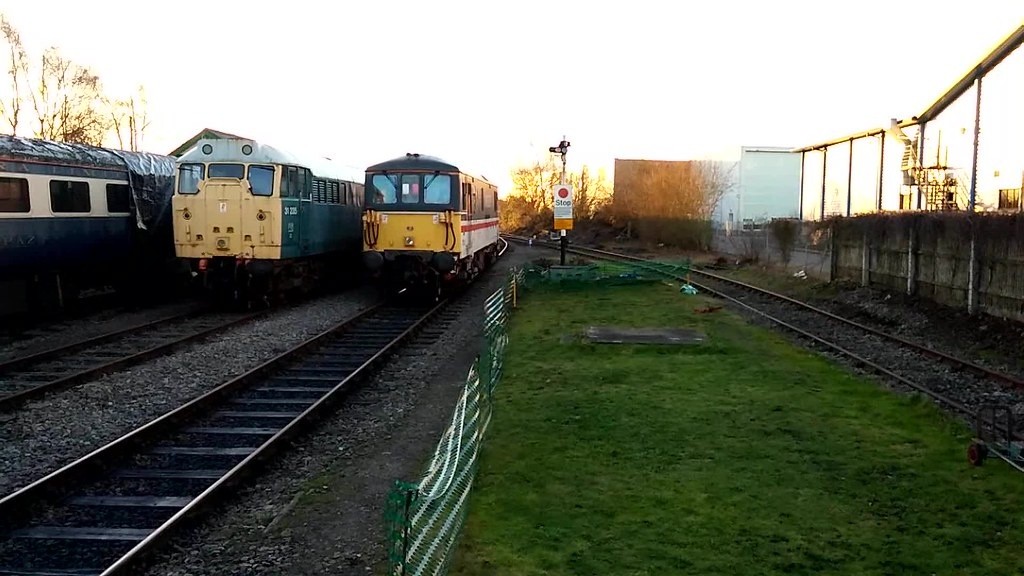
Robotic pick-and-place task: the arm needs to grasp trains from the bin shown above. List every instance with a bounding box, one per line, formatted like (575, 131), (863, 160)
(0, 133), (201, 319)
(363, 153), (500, 294)
(169, 138), (366, 312)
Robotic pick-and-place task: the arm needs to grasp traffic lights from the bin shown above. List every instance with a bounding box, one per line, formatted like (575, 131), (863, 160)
(550, 147), (568, 154)
